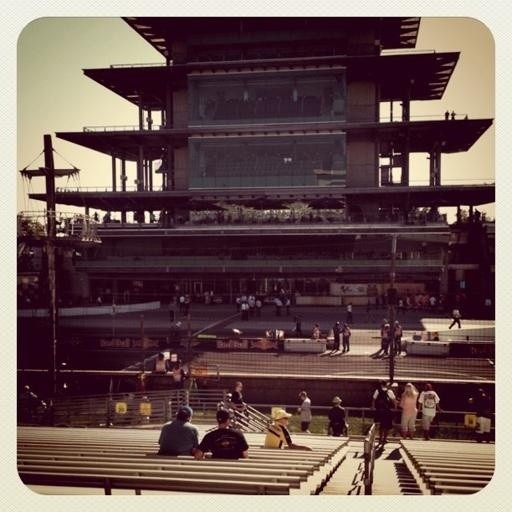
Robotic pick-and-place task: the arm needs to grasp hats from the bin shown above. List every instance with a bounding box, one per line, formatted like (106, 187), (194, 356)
(216, 410), (234, 421)
(178, 406), (193, 419)
(332, 396), (343, 403)
(274, 410), (292, 420)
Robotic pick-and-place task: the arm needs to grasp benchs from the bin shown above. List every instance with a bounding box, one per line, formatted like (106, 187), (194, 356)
(17, 424), (495, 496)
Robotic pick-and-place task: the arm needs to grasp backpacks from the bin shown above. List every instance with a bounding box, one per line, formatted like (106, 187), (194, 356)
(375, 386), (395, 412)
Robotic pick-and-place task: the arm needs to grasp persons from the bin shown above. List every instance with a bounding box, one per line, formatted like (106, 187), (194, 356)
(169, 294), (192, 321)
(328, 395), (349, 436)
(449, 305), (461, 329)
(372, 379), (443, 444)
(236, 294), (291, 321)
(346, 302), (352, 324)
(156, 353), (188, 389)
(312, 324), (320, 339)
(265, 409), (293, 450)
(223, 382), (247, 430)
(376, 294), (443, 314)
(377, 318), (402, 354)
(265, 329), (286, 339)
(156, 405), (199, 456)
(463, 388), (491, 443)
(333, 321), (350, 352)
(194, 410), (249, 460)
(297, 391), (313, 433)
